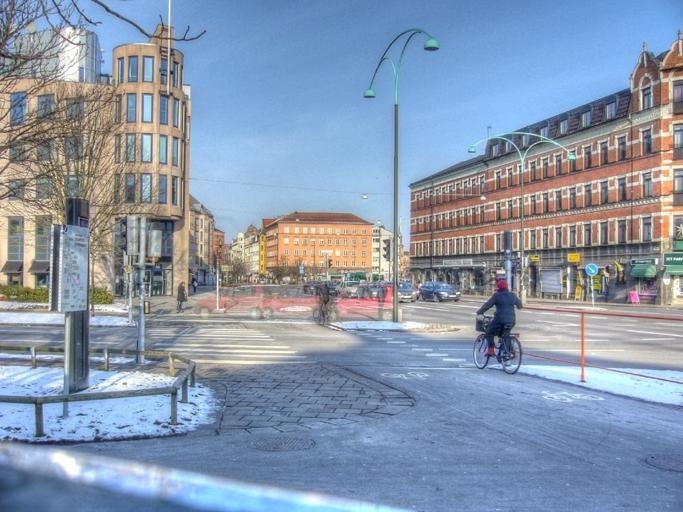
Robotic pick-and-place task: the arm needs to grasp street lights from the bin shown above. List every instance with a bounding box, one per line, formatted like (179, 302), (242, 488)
(467, 132), (576, 304)
(362, 27), (440, 322)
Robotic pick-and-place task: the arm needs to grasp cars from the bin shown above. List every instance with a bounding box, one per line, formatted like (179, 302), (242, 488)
(283, 276), (296, 284)
(304, 281), (461, 303)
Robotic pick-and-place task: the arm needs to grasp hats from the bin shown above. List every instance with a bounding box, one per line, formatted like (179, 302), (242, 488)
(497, 281), (509, 288)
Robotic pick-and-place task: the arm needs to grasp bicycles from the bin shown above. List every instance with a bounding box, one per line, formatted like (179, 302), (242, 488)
(473, 314), (522, 374)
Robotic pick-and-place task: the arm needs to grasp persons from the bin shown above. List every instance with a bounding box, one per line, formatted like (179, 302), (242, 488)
(477, 280), (522, 367)
(191, 281), (197, 292)
(175, 281), (186, 313)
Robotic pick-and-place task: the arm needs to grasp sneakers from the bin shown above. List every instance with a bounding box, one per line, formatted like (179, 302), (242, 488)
(484, 346), (497, 356)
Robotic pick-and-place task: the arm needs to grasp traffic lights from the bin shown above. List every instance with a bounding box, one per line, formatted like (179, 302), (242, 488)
(382, 239), (390, 261)
(329, 259), (332, 268)
